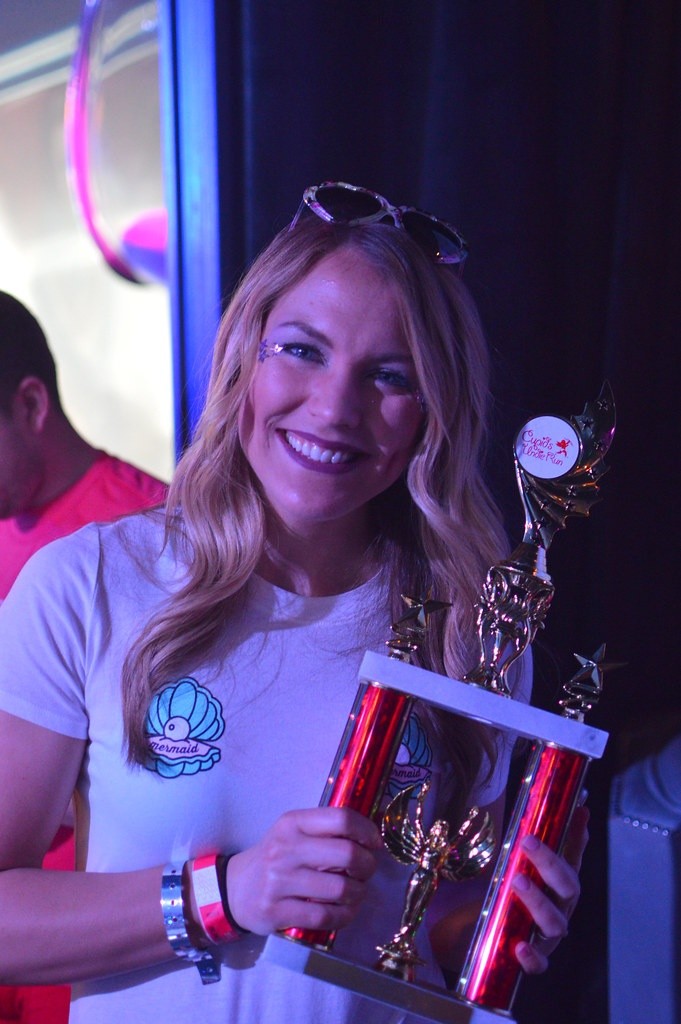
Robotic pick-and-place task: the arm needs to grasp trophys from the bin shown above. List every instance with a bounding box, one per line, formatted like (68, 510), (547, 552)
(256, 386), (619, 1023)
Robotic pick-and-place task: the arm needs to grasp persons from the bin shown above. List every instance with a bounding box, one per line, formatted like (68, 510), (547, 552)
(0, 292), (174, 1023)
(0, 178), (583, 1023)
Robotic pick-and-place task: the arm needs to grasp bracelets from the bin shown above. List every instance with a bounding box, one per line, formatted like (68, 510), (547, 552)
(160, 858), (225, 985)
(191, 839), (250, 948)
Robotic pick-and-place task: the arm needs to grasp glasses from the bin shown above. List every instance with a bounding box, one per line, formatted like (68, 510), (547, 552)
(290, 181), (467, 274)
